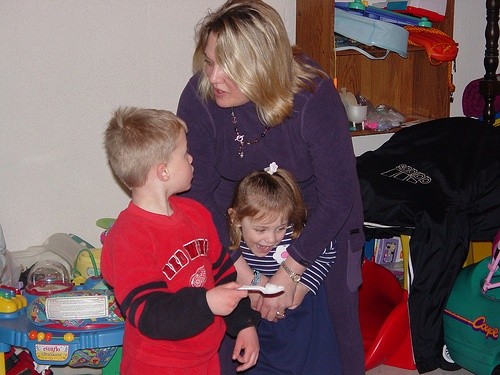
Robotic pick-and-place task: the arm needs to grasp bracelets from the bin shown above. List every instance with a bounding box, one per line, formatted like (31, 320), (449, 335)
(251, 269), (260, 286)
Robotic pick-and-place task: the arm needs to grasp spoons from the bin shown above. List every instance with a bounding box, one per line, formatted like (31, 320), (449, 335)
(237, 284), (284, 294)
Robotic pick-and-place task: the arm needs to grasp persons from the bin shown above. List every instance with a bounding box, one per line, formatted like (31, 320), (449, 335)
(99, 105), (261, 375)
(228, 162), (344, 375)
(177, 0), (365, 375)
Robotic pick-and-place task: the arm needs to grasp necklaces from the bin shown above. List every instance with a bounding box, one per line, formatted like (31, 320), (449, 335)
(229, 105), (269, 159)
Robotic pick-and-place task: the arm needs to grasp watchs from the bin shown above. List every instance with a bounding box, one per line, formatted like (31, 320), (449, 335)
(281, 261), (301, 283)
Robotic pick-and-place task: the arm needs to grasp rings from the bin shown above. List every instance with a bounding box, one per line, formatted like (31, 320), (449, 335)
(282, 314), (286, 318)
(276, 311), (281, 317)
